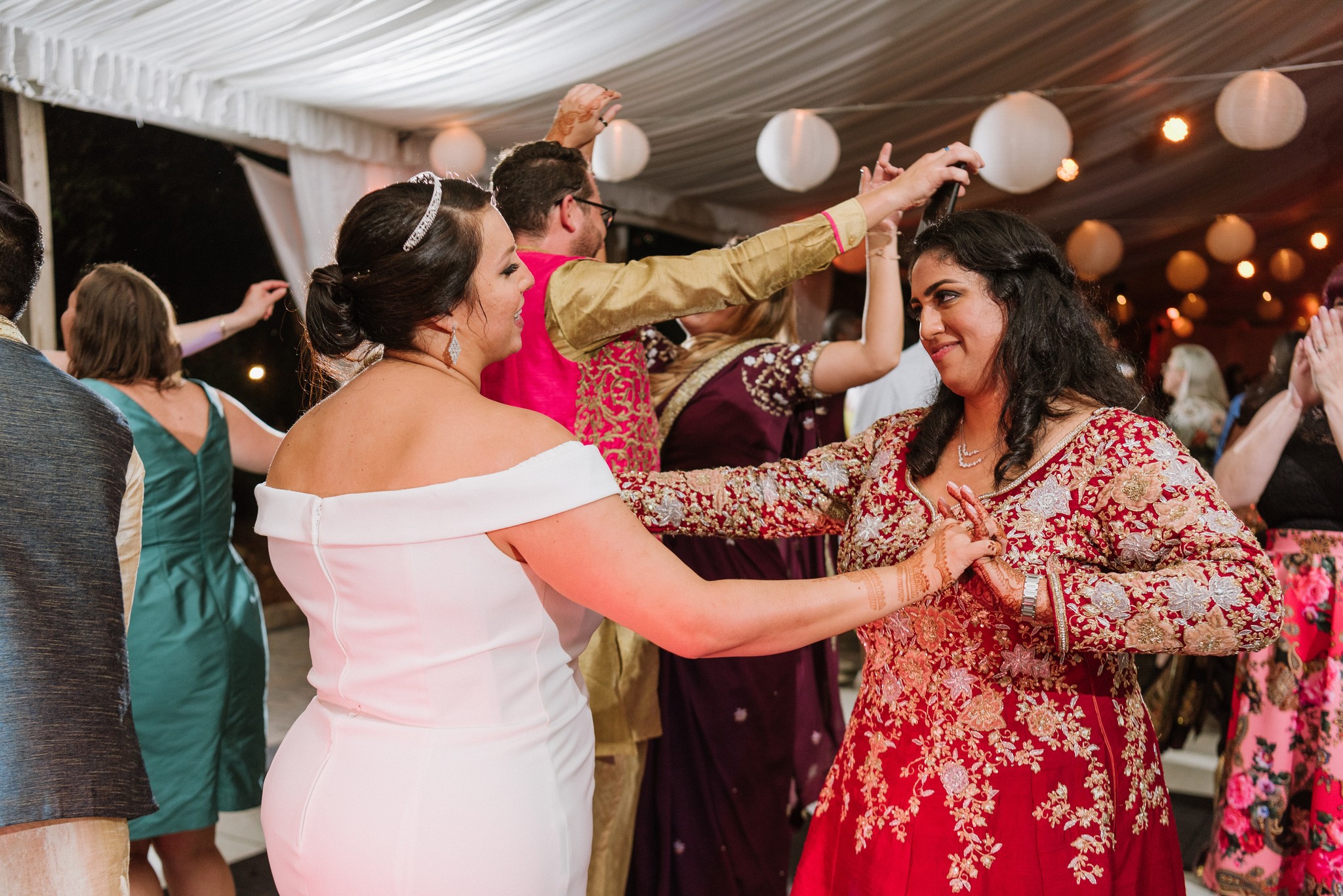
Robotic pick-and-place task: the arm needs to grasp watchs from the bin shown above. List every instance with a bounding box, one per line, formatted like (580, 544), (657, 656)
(1020, 573), (1044, 622)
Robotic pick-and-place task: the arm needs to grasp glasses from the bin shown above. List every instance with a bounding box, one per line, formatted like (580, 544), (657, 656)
(555, 194), (617, 230)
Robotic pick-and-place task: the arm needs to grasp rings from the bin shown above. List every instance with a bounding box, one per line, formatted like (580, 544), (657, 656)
(599, 117), (608, 127)
(602, 86), (608, 92)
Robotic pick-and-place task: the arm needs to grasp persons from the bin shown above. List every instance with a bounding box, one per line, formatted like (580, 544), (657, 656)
(577, 143), (904, 896)
(1205, 264), (1343, 896)
(253, 172), (1003, 896)
(477, 83), (983, 896)
(59, 261), (289, 896)
(616, 213), (1284, 895)
(822, 309), (1305, 448)
(1, 182), (159, 896)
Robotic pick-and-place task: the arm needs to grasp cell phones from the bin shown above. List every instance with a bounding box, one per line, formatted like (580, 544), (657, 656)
(916, 163), (967, 243)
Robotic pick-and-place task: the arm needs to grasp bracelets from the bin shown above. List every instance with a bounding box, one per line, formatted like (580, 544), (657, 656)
(219, 314), (227, 340)
(867, 231), (902, 237)
(865, 247), (902, 260)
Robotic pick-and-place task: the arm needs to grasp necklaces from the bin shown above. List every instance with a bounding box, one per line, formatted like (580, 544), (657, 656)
(959, 411), (1006, 468)
